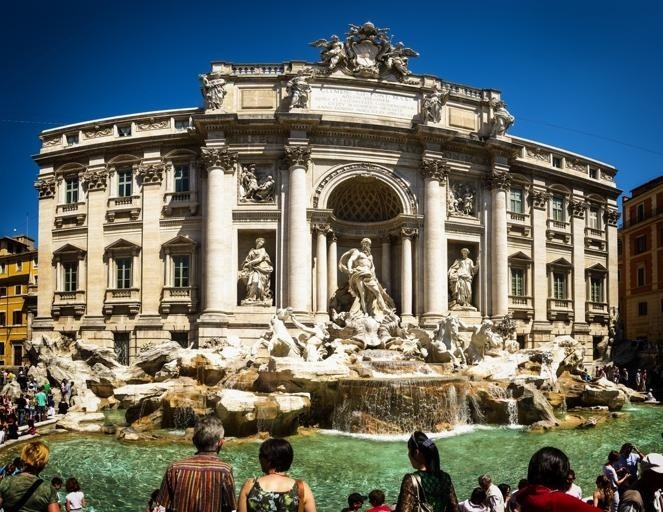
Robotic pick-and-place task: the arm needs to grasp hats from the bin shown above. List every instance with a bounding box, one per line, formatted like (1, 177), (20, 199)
(348, 493), (369, 502)
(641, 452), (663, 473)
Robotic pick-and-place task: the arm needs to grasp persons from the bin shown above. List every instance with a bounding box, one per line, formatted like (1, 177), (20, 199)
(238, 236), (274, 301)
(238, 437), (317, 512)
(146, 490), (161, 511)
(447, 247), (478, 306)
(1, 365), (87, 512)
(238, 164), (275, 200)
(153, 415), (237, 512)
(448, 182), (476, 216)
(338, 237), (391, 316)
(595, 363), (647, 393)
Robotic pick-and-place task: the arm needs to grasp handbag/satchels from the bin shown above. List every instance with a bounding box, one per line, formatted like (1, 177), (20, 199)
(409, 472), (433, 511)
